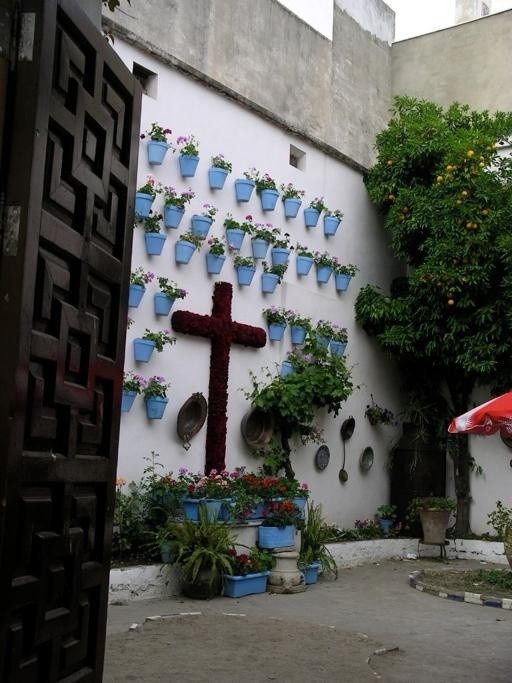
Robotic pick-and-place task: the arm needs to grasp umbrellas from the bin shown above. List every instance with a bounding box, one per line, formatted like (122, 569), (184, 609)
(448, 390), (512, 433)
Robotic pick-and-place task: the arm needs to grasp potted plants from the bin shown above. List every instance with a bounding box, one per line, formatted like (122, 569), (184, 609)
(142, 501), (251, 601)
(409, 496), (454, 543)
(375, 504), (397, 535)
